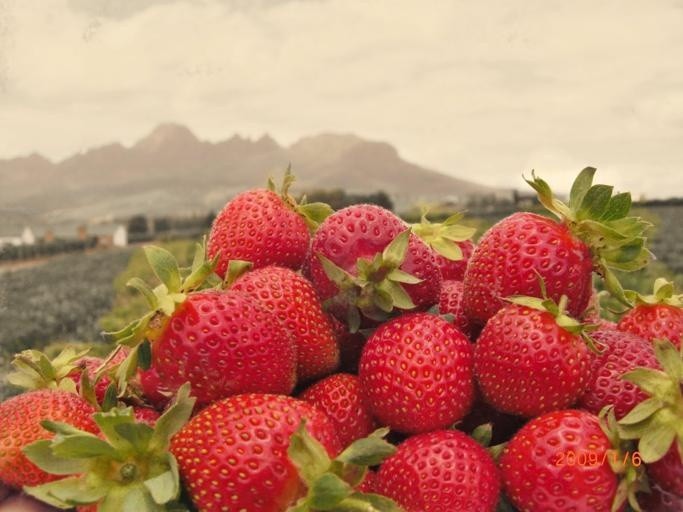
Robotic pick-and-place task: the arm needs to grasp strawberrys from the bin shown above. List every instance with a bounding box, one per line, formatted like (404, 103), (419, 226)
(0, 166), (683, 512)
